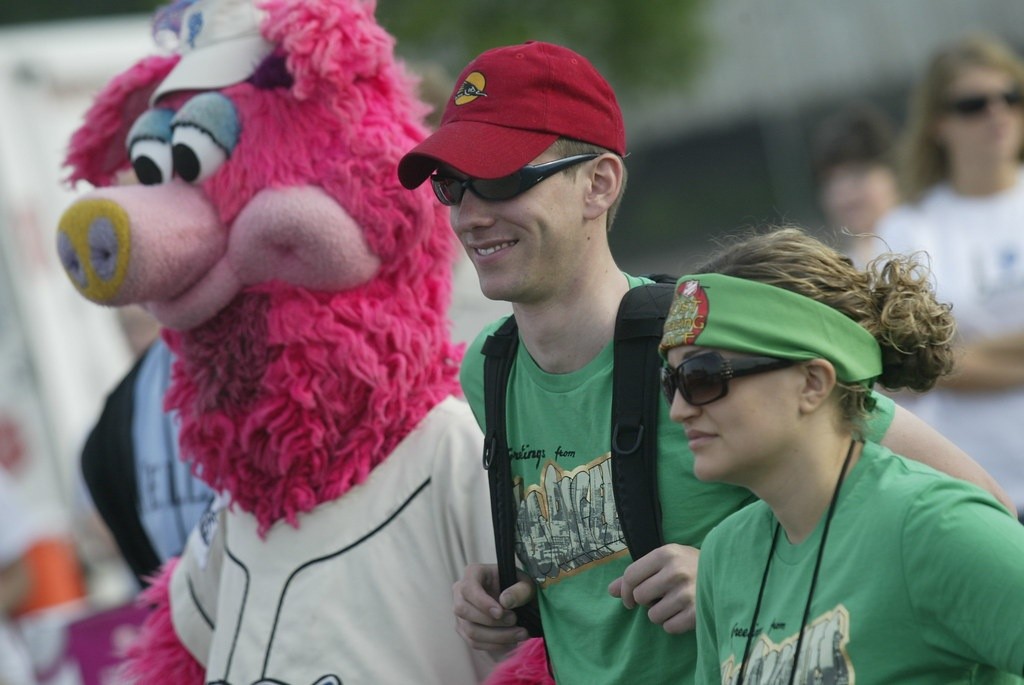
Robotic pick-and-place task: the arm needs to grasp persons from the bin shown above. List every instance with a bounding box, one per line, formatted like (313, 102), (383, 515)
(658, 227), (1024, 685)
(859, 30), (1024, 519)
(808, 118), (898, 240)
(399, 41), (1019, 685)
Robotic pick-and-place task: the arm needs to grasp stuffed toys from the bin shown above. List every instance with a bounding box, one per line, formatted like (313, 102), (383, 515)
(57, 0), (498, 685)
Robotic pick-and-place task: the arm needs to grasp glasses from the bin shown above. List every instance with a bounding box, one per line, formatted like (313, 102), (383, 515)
(664, 350), (795, 406)
(429, 152), (600, 207)
(933, 93), (1024, 118)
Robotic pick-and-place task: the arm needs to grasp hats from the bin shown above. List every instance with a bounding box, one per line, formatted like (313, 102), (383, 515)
(397, 40), (626, 190)
